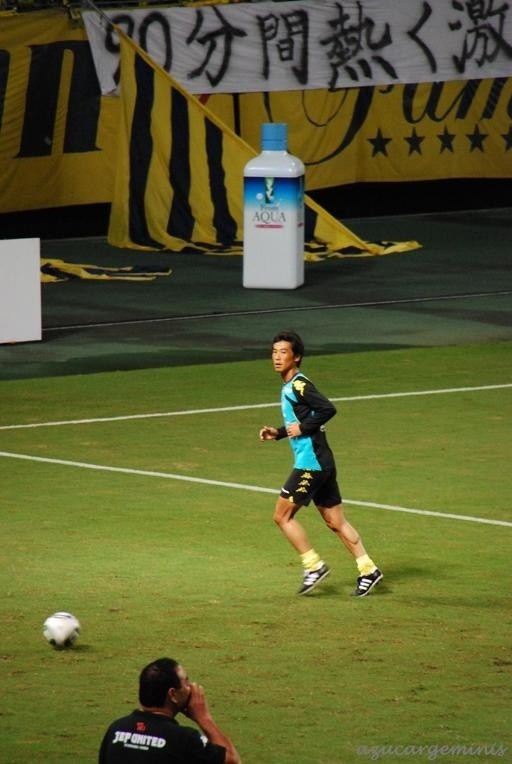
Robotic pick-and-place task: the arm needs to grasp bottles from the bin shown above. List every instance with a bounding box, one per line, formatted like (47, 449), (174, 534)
(241, 121), (306, 293)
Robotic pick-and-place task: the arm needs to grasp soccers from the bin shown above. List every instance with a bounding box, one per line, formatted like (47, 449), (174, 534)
(42, 612), (81, 649)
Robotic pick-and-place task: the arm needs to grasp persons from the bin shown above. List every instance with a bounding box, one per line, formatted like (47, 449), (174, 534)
(97, 655), (246, 764)
(257, 327), (385, 597)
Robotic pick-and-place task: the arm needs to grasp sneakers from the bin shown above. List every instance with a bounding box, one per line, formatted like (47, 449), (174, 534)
(296, 563), (331, 595)
(349, 568), (385, 597)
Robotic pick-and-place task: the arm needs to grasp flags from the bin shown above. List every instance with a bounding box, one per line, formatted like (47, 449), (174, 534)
(104, 24), (423, 267)
